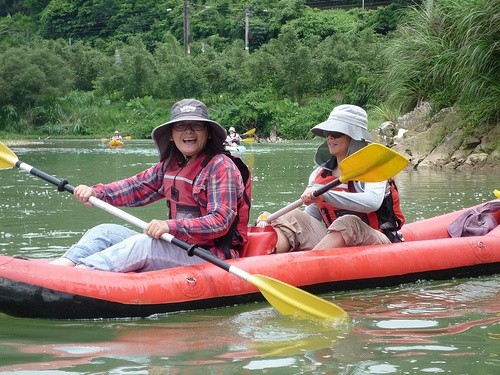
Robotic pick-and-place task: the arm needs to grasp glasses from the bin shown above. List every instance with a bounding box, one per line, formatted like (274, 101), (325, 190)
(322, 130), (345, 138)
(174, 121), (204, 131)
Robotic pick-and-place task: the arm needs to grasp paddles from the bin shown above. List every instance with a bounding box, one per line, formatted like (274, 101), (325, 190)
(101, 135), (132, 143)
(266, 143), (409, 223)
(0, 143), (351, 325)
(223, 128), (256, 145)
(225, 138), (254, 143)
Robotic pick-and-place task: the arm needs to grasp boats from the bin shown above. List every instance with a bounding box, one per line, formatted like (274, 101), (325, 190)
(224, 145), (247, 154)
(108, 139), (124, 148)
(0, 189), (500, 319)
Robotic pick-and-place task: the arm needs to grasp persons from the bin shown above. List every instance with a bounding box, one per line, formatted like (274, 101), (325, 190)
(47, 98), (253, 272)
(223, 127), (242, 145)
(111, 130), (124, 143)
(265, 104), (405, 253)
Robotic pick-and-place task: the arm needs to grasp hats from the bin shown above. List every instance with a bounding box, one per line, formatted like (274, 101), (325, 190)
(228, 127), (236, 132)
(115, 131), (119, 133)
(310, 105), (373, 144)
(151, 99), (227, 152)
